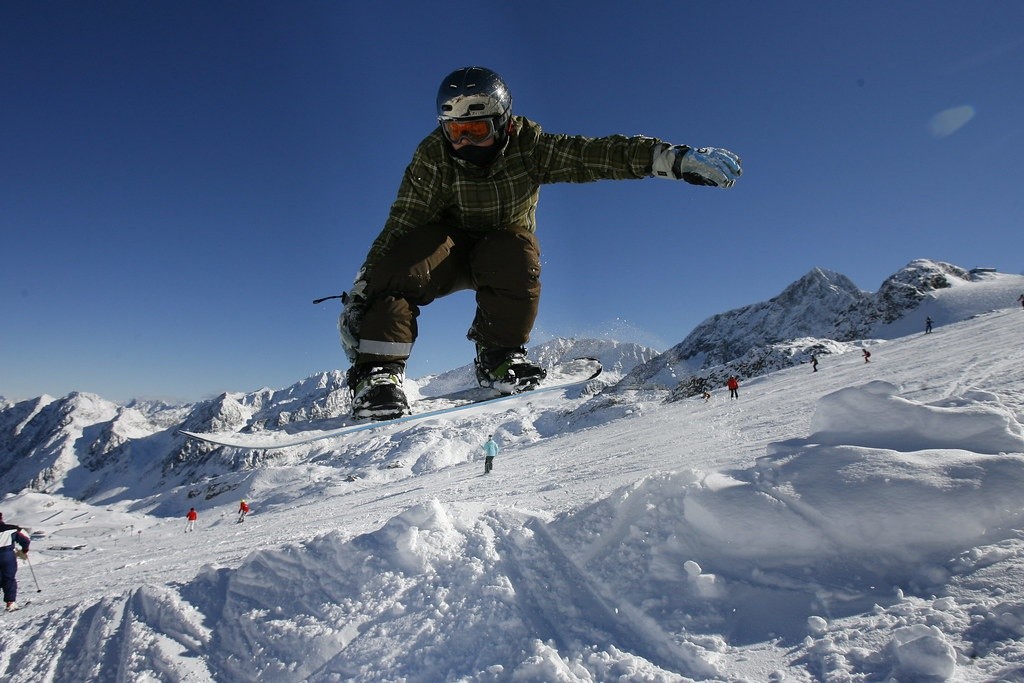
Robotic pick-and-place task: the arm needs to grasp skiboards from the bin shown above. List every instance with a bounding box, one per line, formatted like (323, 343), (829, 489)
(0, 601), (31, 616)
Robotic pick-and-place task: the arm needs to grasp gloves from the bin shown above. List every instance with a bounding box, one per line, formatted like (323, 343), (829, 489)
(651, 142), (743, 188)
(338, 283), (365, 364)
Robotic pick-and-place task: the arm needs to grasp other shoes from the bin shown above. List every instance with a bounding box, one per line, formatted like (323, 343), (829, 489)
(7, 602), (18, 609)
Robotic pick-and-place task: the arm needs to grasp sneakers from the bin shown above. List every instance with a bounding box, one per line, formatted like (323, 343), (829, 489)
(474, 345), (546, 385)
(347, 366), (406, 419)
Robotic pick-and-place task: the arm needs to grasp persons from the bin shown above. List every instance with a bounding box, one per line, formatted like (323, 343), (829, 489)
(237, 499), (248, 523)
(480, 434), (498, 475)
(184, 507), (197, 533)
(701, 316), (934, 402)
(1017, 294), (1024, 310)
(339, 68), (743, 421)
(0, 512), (31, 611)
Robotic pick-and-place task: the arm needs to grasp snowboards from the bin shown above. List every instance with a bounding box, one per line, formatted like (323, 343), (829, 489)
(176, 356), (604, 448)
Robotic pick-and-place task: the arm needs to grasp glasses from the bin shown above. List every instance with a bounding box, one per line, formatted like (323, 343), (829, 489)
(438, 97), (511, 146)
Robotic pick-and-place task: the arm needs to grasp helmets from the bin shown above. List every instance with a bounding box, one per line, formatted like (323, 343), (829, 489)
(241, 499), (246, 502)
(436, 67), (513, 182)
